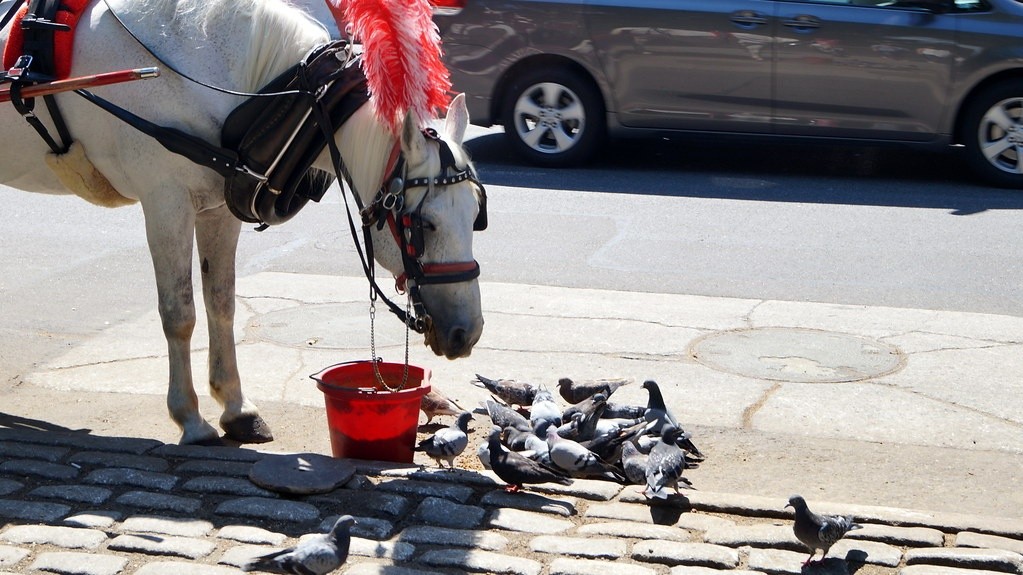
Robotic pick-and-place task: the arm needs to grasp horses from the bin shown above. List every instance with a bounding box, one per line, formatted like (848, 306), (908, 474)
(0, 0), (485, 448)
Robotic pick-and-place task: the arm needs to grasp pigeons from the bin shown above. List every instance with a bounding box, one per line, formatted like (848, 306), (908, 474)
(411, 373), (707, 498)
(217, 515), (359, 575)
(784, 495), (863, 570)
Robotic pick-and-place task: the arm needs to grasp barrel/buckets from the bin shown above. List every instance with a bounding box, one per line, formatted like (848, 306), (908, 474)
(309, 356), (432, 463)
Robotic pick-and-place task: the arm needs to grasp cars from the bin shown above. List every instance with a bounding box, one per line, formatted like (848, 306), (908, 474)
(423, 0), (1022, 191)
